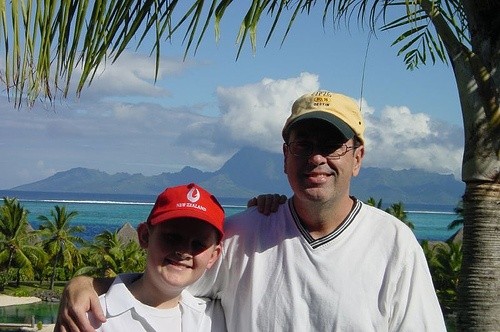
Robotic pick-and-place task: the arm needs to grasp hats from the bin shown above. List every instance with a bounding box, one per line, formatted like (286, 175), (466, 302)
(282, 90), (365, 145)
(149, 183), (225, 245)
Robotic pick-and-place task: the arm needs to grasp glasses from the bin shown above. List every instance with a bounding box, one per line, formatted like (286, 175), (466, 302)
(285, 142), (357, 156)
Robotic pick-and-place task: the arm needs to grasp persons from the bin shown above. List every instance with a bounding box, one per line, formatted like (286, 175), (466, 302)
(53, 88), (448, 332)
(78, 180), (288, 332)
